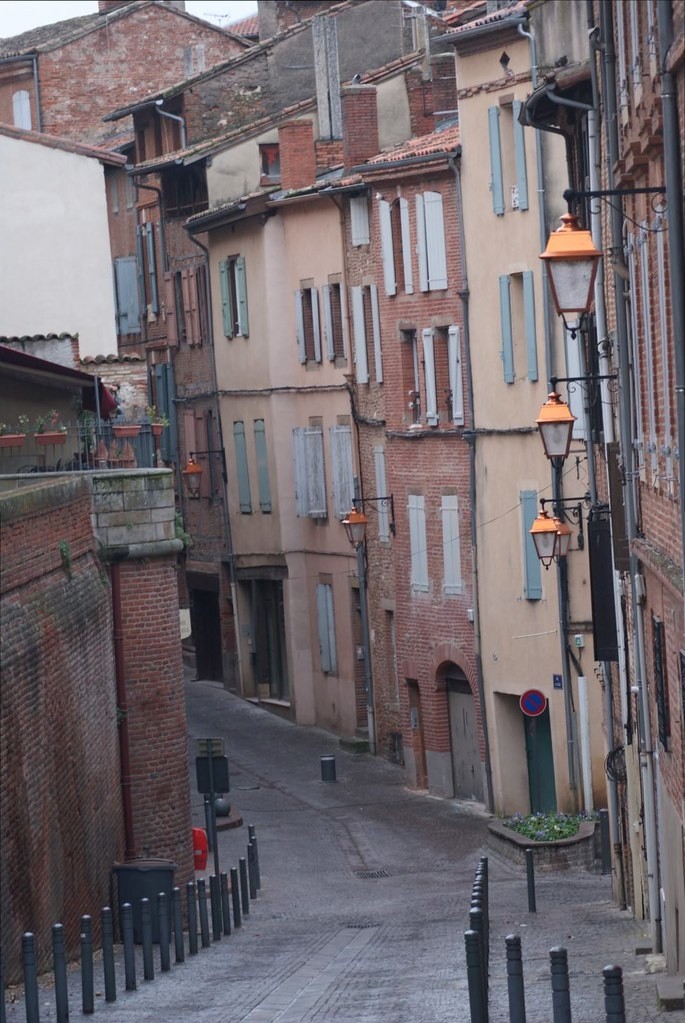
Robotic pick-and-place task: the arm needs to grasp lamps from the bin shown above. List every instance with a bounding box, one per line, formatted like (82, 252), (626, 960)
(535, 369), (615, 468)
(182, 449), (225, 498)
(532, 495), (591, 568)
(541, 187), (665, 339)
(340, 494), (393, 551)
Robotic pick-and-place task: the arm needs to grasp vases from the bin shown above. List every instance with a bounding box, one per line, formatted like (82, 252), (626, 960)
(33, 432), (67, 445)
(0, 434), (26, 447)
(111, 425), (140, 437)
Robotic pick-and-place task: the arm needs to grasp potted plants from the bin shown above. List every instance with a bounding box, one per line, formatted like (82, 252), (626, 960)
(148, 407), (170, 434)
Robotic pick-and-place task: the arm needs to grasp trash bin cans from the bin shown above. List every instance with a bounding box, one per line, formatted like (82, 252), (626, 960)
(113, 860), (179, 944)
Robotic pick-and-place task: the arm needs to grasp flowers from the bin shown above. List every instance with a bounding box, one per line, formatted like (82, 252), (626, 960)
(0, 414), (30, 436)
(35, 410), (66, 434)
